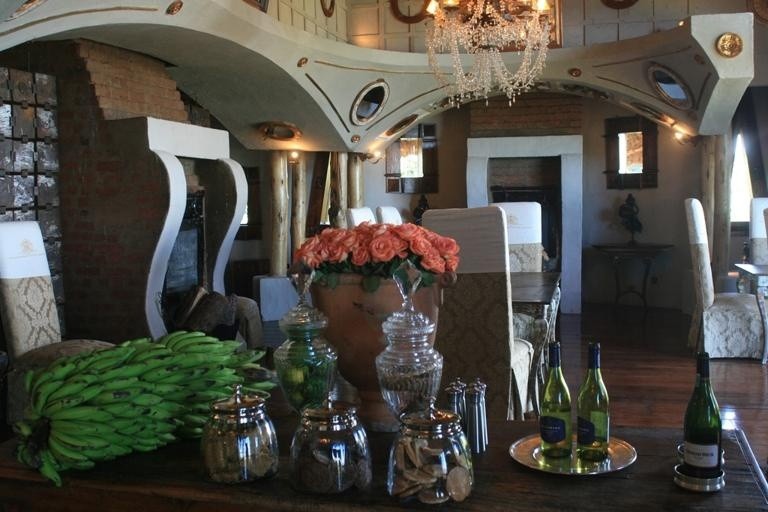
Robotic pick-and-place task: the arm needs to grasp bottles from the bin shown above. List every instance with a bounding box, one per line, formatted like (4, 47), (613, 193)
(449, 376), (467, 418)
(683, 352), (722, 478)
(465, 383), (487, 457)
(468, 376), (489, 448)
(576, 341), (611, 462)
(539, 341), (573, 459)
(445, 381), (468, 440)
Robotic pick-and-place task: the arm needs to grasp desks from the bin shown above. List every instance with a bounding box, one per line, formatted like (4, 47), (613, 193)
(0, 425), (768, 512)
(592, 241), (676, 315)
(732, 261), (768, 275)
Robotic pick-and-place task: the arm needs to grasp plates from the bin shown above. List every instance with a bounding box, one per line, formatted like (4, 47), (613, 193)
(508, 428), (638, 477)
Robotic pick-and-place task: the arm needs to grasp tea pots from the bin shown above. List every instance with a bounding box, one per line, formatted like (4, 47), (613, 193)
(292, 398), (371, 495)
(204, 384), (277, 483)
(389, 395), (471, 506)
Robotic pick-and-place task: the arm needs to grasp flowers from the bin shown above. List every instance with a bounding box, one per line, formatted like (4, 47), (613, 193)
(292, 222), (460, 275)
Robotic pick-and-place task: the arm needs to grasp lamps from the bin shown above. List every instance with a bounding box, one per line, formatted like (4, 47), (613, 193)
(424, 1), (551, 106)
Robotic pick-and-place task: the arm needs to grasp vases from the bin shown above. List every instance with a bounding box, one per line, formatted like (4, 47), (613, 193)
(310, 275), (458, 422)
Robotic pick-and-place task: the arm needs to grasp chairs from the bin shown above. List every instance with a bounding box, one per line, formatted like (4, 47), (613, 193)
(378, 205), (404, 225)
(684, 197), (763, 361)
(490, 199), (559, 313)
(749, 197), (768, 365)
(345, 205), (377, 227)
(422, 206), (539, 422)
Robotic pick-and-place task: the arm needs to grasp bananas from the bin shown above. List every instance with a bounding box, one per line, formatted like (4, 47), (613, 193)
(11, 328), (281, 488)
